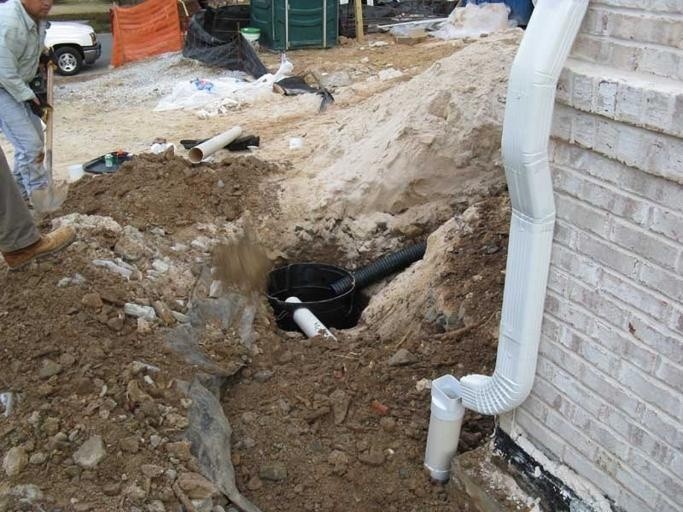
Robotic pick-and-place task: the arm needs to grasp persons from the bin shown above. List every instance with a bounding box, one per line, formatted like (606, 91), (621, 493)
(0, 143), (76, 270)
(0, 0), (54, 208)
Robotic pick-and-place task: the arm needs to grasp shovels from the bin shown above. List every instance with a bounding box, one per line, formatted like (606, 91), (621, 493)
(31, 66), (69, 215)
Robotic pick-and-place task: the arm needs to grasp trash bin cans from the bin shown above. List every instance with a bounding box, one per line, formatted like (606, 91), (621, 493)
(240, 27), (261, 54)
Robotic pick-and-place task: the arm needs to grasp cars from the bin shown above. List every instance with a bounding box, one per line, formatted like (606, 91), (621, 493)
(39, 19), (102, 76)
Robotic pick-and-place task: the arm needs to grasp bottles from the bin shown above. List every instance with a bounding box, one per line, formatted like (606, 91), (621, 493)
(105, 153), (114, 168)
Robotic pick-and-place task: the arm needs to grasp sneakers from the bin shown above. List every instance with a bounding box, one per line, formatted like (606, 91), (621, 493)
(3, 226), (76, 267)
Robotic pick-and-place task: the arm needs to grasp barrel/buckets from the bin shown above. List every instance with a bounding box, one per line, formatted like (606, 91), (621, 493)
(241, 28), (261, 43)
